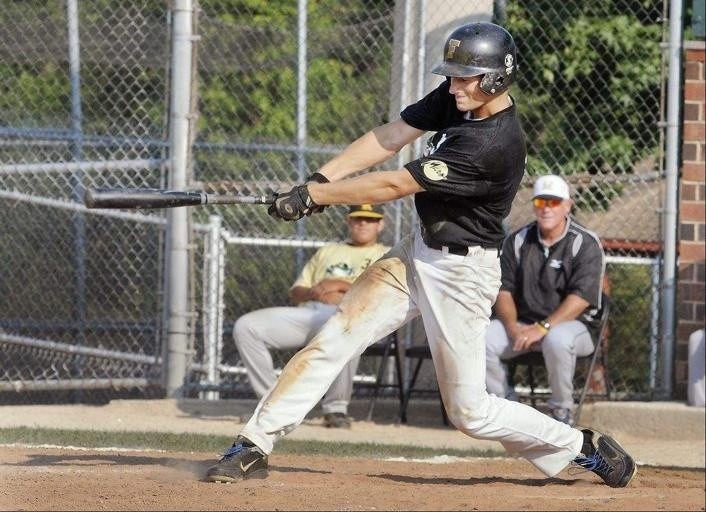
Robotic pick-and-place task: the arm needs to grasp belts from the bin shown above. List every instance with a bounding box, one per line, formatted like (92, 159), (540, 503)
(420, 223), (468, 256)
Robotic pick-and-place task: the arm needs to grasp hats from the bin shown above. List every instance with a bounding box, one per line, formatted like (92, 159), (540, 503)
(531, 174), (570, 201)
(347, 203), (384, 219)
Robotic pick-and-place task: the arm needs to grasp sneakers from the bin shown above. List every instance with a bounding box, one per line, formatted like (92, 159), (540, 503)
(322, 413), (350, 428)
(573, 427), (637, 487)
(206, 438), (269, 484)
(547, 408), (576, 427)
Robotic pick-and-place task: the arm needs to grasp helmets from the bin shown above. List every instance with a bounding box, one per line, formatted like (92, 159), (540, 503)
(431, 21), (516, 96)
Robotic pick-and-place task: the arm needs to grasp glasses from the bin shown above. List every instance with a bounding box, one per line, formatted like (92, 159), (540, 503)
(532, 197), (562, 207)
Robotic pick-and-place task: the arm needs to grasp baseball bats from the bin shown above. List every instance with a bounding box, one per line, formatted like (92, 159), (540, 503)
(84, 188), (280, 209)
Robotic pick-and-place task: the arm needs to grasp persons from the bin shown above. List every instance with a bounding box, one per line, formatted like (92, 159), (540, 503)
(233, 200), (397, 429)
(481, 175), (609, 427)
(205, 22), (638, 487)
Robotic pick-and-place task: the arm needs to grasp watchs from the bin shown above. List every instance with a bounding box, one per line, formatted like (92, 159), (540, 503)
(534, 317), (550, 334)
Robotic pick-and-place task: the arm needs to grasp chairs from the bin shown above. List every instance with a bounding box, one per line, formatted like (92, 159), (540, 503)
(502, 292), (612, 427)
(350, 329), (407, 425)
(396, 337), (450, 427)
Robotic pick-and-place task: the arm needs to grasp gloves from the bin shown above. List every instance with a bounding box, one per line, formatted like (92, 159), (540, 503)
(268, 173), (331, 221)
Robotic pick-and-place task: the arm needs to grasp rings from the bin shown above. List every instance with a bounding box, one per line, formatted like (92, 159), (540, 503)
(523, 335), (529, 341)
